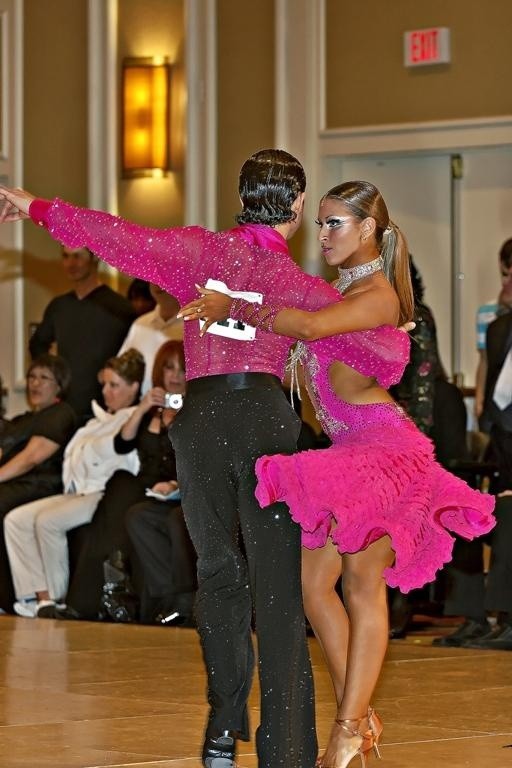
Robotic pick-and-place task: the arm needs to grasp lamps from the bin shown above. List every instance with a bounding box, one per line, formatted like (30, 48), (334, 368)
(119, 55), (172, 177)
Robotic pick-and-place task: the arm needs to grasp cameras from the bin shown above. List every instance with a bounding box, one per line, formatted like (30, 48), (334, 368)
(162, 392), (184, 411)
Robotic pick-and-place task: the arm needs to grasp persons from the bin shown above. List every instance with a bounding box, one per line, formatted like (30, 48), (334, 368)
(174, 179), (499, 767)
(0, 233), (512, 650)
(0, 146), (415, 768)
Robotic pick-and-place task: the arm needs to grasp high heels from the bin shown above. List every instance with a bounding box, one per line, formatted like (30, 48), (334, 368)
(366, 707), (384, 758)
(317, 717), (375, 767)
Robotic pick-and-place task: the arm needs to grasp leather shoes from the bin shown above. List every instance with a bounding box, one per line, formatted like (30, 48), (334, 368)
(464, 623), (512, 649)
(432, 619), (491, 646)
(203, 725), (237, 768)
(13, 600), (66, 617)
